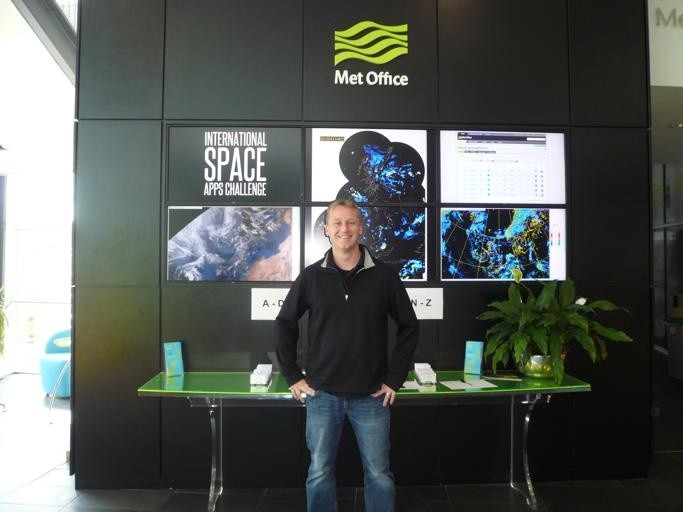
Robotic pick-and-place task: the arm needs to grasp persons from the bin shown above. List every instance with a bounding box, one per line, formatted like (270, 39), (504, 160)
(275, 199), (418, 508)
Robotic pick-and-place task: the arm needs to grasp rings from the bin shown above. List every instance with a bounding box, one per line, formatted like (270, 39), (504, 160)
(386, 393), (390, 395)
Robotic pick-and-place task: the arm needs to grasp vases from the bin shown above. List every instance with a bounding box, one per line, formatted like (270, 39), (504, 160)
(512, 350), (566, 379)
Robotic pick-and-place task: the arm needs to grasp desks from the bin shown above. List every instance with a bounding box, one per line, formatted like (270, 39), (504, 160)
(136, 369), (592, 512)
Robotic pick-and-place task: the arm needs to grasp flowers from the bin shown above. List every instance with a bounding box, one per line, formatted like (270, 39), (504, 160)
(475, 268), (634, 386)
